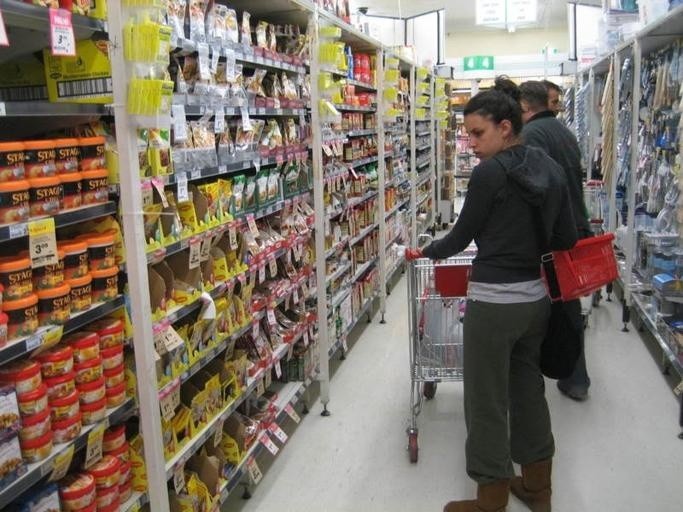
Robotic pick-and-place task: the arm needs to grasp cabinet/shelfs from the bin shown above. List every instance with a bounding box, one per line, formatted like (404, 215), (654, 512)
(0, 0), (457, 512)
(558, 5), (683, 426)
(449, 87), (480, 197)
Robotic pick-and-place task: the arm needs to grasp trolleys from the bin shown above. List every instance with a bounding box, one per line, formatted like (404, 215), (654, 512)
(407, 234), (479, 463)
(580, 181), (605, 329)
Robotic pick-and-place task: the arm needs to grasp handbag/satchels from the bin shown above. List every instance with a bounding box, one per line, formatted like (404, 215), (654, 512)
(539, 301), (585, 380)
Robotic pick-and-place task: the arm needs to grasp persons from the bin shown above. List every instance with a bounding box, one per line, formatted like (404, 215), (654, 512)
(414, 74), (580, 512)
(540, 78), (562, 117)
(514, 81), (593, 400)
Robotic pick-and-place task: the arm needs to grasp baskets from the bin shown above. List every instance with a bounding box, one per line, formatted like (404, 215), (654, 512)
(548, 233), (621, 301)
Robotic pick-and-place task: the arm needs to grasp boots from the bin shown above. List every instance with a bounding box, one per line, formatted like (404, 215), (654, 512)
(441, 480), (510, 512)
(510, 456), (556, 512)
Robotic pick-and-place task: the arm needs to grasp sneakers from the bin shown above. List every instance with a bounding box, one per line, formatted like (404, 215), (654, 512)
(556, 377), (592, 405)
